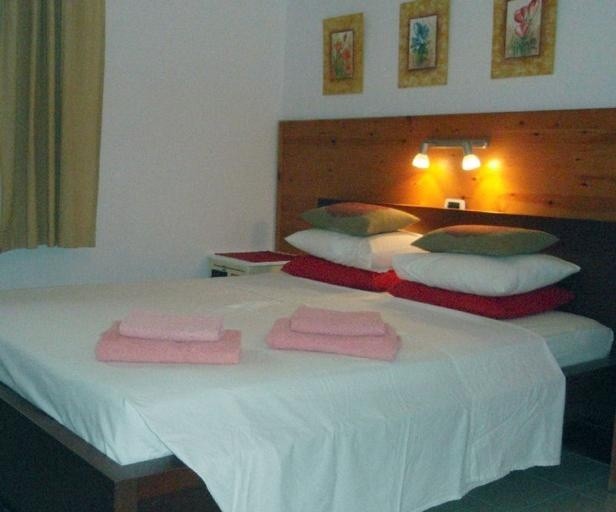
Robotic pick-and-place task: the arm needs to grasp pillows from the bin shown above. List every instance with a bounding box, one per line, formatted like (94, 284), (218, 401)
(300, 200), (421, 237)
(412, 224), (560, 258)
(281, 254), (395, 293)
(388, 276), (582, 319)
(390, 249), (582, 298)
(283, 228), (426, 273)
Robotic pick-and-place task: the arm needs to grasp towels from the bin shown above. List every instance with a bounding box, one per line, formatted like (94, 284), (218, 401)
(265, 304), (404, 363)
(93, 306), (244, 367)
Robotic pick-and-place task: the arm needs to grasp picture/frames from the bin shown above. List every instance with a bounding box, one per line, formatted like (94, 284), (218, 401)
(397, 1), (450, 89)
(490, 1), (557, 80)
(322, 11), (364, 95)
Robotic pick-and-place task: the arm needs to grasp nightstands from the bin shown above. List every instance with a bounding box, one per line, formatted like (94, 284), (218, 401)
(209, 249), (297, 276)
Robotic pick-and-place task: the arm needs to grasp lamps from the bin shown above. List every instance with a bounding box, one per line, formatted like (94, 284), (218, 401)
(412, 136), (488, 173)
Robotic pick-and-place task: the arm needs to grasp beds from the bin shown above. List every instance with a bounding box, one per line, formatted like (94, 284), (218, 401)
(1, 196), (616, 512)
